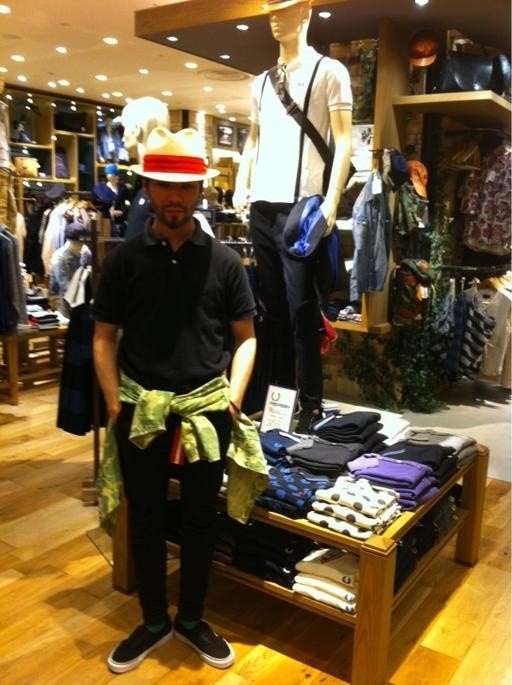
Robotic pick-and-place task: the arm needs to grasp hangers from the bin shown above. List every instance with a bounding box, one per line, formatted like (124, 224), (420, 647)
(436, 125), (512, 172)
(442, 270), (511, 308)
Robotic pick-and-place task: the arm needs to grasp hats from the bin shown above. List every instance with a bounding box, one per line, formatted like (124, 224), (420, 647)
(268, 0), (300, 12)
(104, 165), (117, 174)
(407, 160), (428, 199)
(383, 147), (410, 193)
(409, 31), (439, 67)
(45, 183), (63, 198)
(64, 222), (87, 240)
(130, 128), (221, 183)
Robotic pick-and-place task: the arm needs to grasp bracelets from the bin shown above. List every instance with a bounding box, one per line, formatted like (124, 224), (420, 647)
(228, 397), (241, 415)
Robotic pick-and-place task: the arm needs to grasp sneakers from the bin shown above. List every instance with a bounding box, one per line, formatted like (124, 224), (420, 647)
(175, 614), (235, 669)
(295, 406), (322, 434)
(108, 614), (173, 674)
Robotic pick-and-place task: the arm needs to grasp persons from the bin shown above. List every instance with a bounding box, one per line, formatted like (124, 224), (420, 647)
(93, 163), (133, 221)
(47, 223), (93, 319)
(231, 0), (353, 435)
(91, 125), (260, 676)
(121, 95), (173, 241)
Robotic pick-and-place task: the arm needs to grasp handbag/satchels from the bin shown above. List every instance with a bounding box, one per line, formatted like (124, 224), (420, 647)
(323, 154), (356, 197)
(46, 290), (60, 309)
(388, 258), (430, 327)
(393, 182), (430, 236)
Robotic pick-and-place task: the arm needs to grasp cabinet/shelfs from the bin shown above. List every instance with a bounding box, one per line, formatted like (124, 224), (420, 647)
(109, 406), (491, 685)
(202, 113), (250, 265)
(0, 84), (145, 407)
